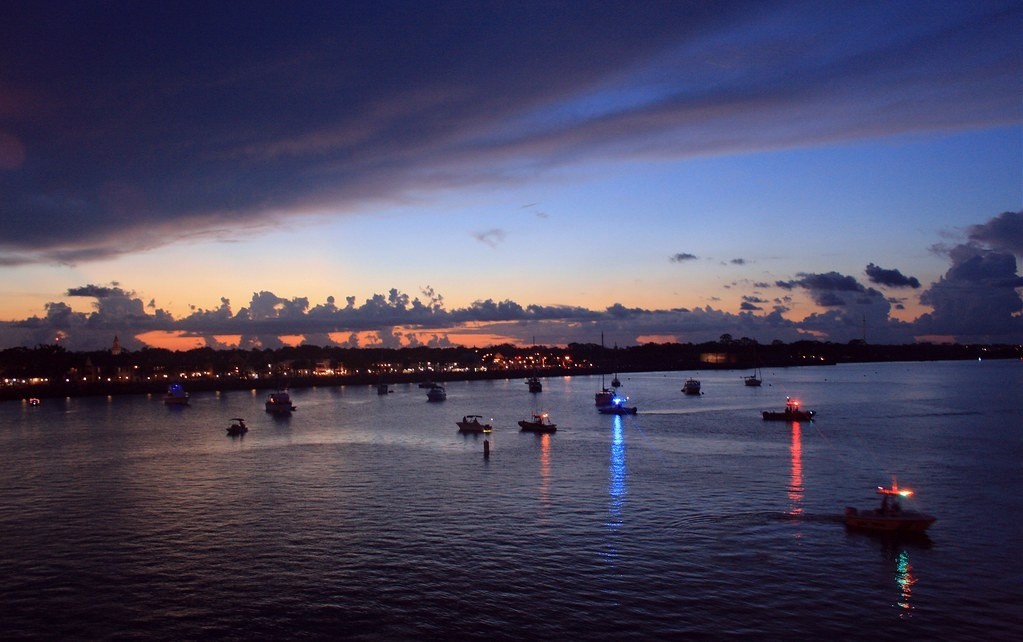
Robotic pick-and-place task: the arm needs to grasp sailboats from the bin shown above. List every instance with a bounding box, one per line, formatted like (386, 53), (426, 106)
(611, 340), (621, 387)
(522, 335), (543, 393)
(745, 336), (762, 387)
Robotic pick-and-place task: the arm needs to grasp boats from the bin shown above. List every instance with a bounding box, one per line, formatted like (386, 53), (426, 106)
(418, 382), (448, 403)
(518, 412), (557, 433)
(763, 396), (817, 423)
(378, 384), (389, 395)
(450, 412), (493, 434)
(162, 384), (190, 404)
(597, 397), (638, 415)
(226, 418), (249, 435)
(843, 493), (940, 535)
(265, 380), (293, 418)
(595, 330), (616, 406)
(681, 376), (701, 395)
